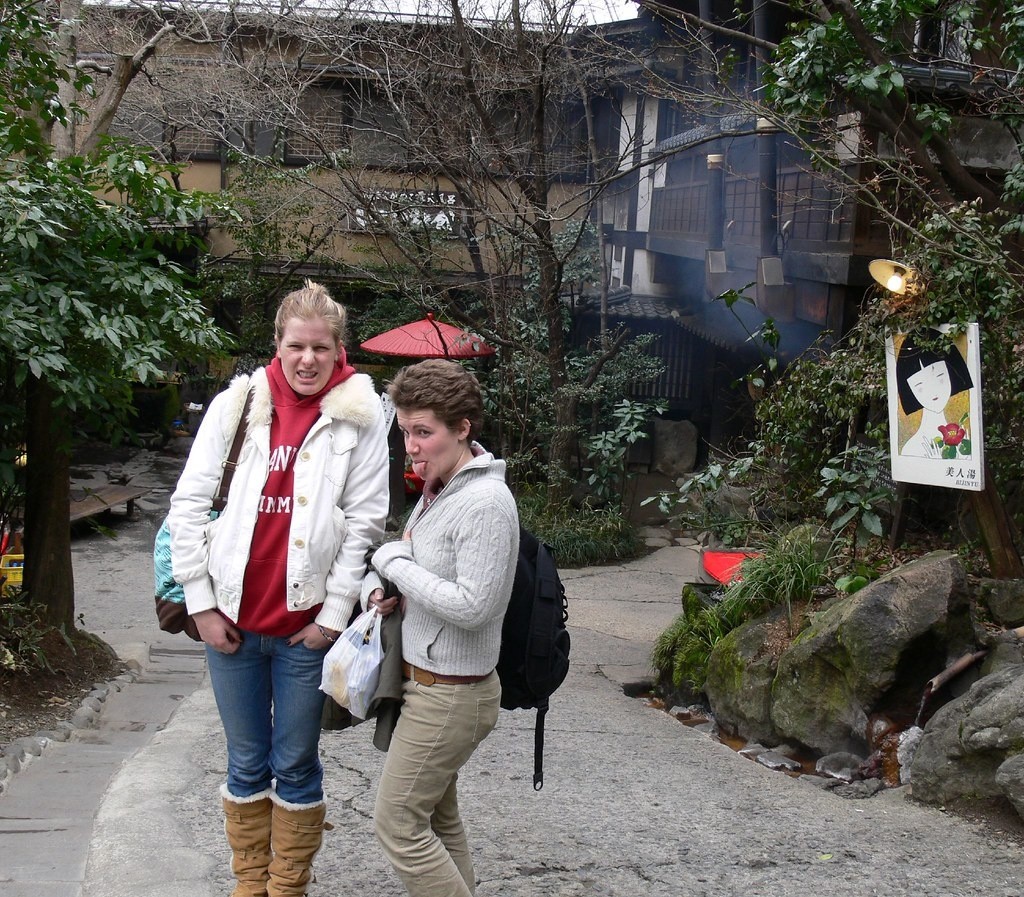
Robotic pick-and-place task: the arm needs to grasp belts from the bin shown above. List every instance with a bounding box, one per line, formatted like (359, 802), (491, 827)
(397, 660), (494, 687)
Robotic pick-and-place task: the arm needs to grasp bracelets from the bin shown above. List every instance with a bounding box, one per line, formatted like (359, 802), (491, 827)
(317, 626), (340, 643)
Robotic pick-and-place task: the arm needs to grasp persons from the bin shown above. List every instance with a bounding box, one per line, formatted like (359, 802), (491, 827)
(364, 356), (519, 897)
(167, 279), (390, 897)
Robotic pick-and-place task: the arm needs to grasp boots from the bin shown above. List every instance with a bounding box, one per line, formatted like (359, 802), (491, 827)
(220, 785), (332, 896)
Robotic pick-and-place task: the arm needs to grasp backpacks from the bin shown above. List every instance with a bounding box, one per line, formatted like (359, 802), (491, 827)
(497, 521), (571, 713)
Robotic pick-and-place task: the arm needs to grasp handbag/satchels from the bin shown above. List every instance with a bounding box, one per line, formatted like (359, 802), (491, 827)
(317, 604), (384, 722)
(155, 512), (218, 643)
